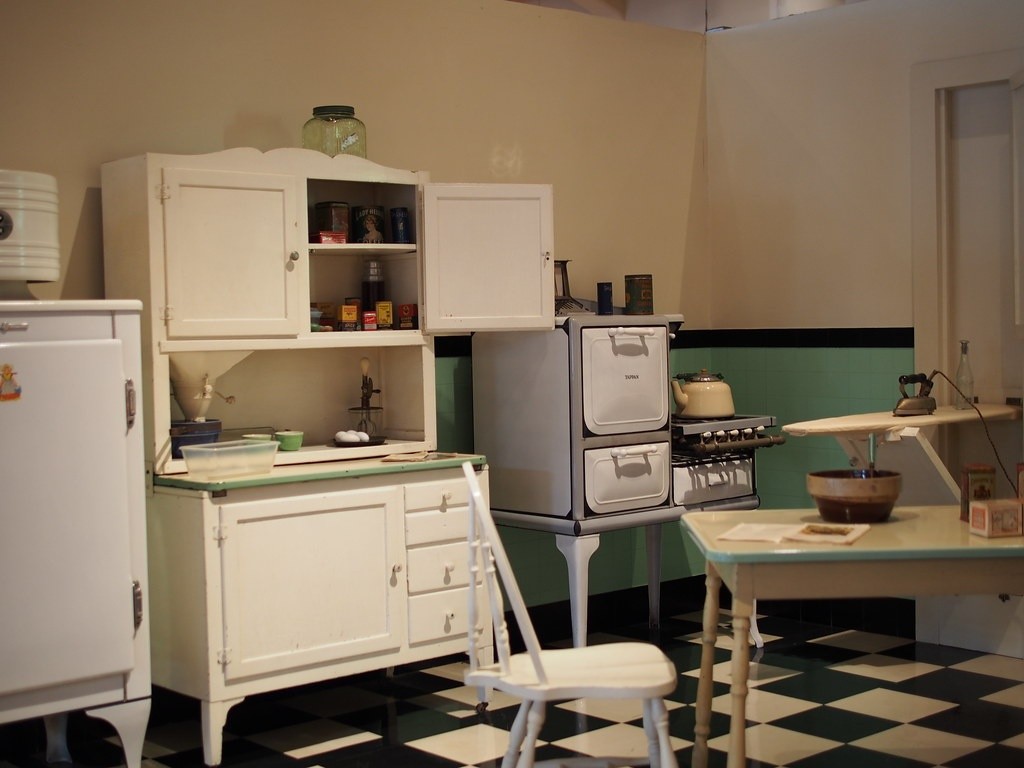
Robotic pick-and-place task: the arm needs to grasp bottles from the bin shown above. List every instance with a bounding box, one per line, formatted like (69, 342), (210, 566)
(303, 106), (367, 161)
(955, 340), (974, 410)
(362, 261), (387, 312)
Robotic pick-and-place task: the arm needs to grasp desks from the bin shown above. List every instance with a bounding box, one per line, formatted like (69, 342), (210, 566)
(680, 505), (1024, 768)
(783, 403), (1023, 502)
(490, 497), (763, 650)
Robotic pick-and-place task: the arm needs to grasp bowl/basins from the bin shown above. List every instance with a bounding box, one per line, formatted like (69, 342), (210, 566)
(807, 470), (902, 523)
(274, 431), (304, 451)
(242, 434), (271, 442)
(171, 420), (222, 460)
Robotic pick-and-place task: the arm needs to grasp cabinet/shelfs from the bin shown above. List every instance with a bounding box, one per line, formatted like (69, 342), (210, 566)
(472, 315), (756, 522)
(100, 147), (555, 766)
(0, 297), (151, 768)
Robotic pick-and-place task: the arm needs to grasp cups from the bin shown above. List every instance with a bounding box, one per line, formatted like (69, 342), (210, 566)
(391, 208), (409, 243)
(597, 282), (613, 315)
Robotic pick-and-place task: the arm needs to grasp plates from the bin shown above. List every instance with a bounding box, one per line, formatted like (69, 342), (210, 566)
(333, 436), (387, 447)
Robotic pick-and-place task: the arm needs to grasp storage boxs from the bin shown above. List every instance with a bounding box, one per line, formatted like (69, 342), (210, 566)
(310, 301), (417, 331)
(969, 499), (1022, 539)
(308, 231), (348, 244)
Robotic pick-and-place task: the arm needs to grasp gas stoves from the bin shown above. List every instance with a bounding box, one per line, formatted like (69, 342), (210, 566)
(671, 412), (786, 460)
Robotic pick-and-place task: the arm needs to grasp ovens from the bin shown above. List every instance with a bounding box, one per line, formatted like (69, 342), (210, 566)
(472, 315), (673, 536)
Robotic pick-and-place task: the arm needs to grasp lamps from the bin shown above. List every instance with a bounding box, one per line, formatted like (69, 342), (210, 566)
(0, 170), (60, 301)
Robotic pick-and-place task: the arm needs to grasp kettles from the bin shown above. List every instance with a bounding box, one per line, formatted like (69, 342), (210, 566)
(672, 369), (735, 420)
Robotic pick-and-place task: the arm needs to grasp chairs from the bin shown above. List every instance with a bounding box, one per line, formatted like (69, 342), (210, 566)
(462, 462), (680, 768)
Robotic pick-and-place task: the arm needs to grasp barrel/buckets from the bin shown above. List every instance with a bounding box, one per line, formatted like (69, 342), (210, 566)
(351, 206), (384, 243)
(625, 274), (653, 315)
